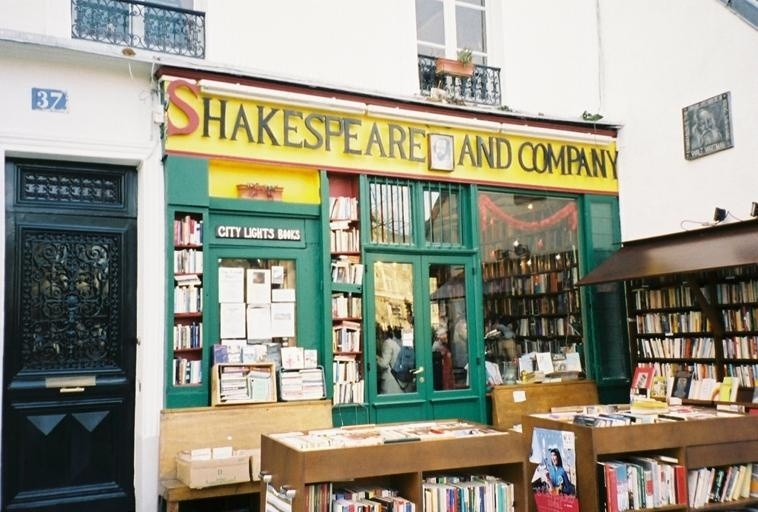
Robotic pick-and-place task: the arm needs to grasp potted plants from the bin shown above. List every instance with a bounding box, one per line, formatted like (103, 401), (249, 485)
(435, 46), (475, 77)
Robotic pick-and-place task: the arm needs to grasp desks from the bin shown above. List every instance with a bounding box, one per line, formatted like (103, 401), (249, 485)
(160, 478), (261, 512)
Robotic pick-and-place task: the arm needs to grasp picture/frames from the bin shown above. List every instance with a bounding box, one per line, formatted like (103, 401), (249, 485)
(631, 367), (655, 390)
(428, 132), (455, 172)
(682, 92), (734, 161)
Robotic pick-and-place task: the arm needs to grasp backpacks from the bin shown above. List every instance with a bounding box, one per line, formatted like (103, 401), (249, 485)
(391, 346), (414, 389)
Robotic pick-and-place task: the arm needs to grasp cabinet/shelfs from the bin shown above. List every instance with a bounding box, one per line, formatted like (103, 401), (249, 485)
(483, 251), (583, 362)
(259, 419), (530, 512)
(521, 407), (758, 511)
(173, 242), (203, 353)
(328, 173), (362, 357)
(627, 264), (758, 400)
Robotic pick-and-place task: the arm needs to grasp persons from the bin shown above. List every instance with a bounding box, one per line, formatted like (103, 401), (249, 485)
(692, 108), (722, 150)
(377, 332), (410, 395)
(545, 448), (574, 495)
(430, 326), (457, 391)
(430, 138), (452, 168)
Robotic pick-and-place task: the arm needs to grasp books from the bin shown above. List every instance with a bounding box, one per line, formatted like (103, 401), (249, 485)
(685, 463), (758, 509)
(271, 422), (510, 453)
(179, 447), (236, 458)
(529, 399), (747, 427)
(212, 343), (326, 405)
(173, 215), (205, 386)
(479, 210), (584, 385)
(422, 471), (515, 511)
(596, 453), (686, 512)
(329, 196), (365, 406)
(306, 482), (415, 512)
(633, 275), (758, 403)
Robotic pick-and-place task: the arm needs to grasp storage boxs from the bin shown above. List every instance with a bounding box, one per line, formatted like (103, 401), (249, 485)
(175, 454), (251, 490)
(277, 365), (327, 400)
(211, 362), (279, 405)
(251, 451), (262, 481)
